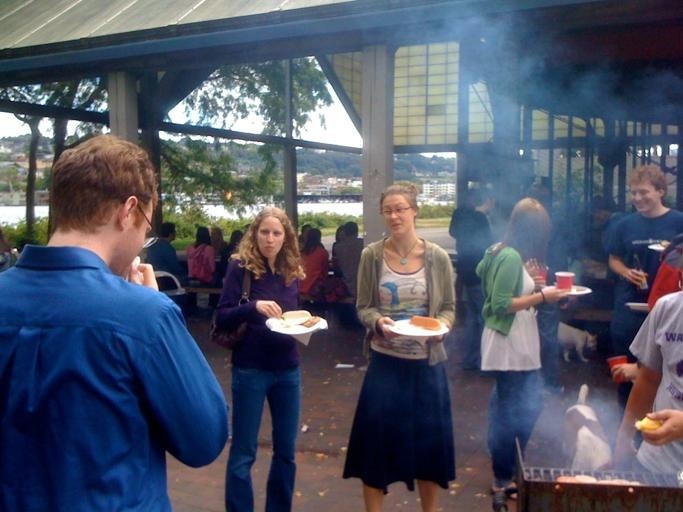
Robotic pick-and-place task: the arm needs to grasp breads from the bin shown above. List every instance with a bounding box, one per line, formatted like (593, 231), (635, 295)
(409, 315), (442, 331)
(282, 310), (312, 326)
(633, 416), (663, 432)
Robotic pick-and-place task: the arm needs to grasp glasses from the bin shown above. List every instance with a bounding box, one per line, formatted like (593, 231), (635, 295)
(379, 207), (411, 215)
(120, 193), (161, 248)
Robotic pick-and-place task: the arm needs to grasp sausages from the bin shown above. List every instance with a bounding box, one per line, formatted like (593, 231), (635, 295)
(304, 315), (320, 328)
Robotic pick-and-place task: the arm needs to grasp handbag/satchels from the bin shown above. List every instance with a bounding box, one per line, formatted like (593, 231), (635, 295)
(208, 297), (250, 349)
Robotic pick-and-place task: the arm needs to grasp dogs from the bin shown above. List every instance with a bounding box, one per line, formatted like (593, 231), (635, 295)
(557, 321), (597, 363)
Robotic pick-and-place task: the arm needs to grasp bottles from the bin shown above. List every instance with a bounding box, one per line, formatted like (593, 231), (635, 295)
(632, 255), (650, 291)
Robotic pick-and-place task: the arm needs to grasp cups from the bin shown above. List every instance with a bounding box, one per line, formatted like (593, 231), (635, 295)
(554, 270), (575, 292)
(607, 357), (628, 383)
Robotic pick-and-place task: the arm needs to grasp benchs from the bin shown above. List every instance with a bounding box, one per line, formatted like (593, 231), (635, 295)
(300, 292), (356, 315)
(570, 309), (612, 332)
(184, 284), (222, 307)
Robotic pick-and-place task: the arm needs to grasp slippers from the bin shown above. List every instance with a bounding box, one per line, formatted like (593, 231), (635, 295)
(490, 486), (518, 501)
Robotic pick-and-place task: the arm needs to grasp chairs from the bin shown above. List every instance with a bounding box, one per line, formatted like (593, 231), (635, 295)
(154, 270), (186, 295)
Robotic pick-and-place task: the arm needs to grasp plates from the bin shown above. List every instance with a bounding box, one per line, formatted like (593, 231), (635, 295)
(626, 302), (650, 311)
(648, 243), (671, 253)
(542, 285), (593, 296)
(264, 316), (326, 335)
(389, 318), (450, 338)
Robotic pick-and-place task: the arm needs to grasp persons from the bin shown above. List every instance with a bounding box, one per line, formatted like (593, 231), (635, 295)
(445, 176), (631, 373)
(614, 290), (683, 474)
(475, 197), (564, 512)
(211, 206), (306, 512)
(0, 135), (227, 512)
(144, 221), (366, 347)
(342, 178), (457, 512)
(605, 167), (683, 449)
